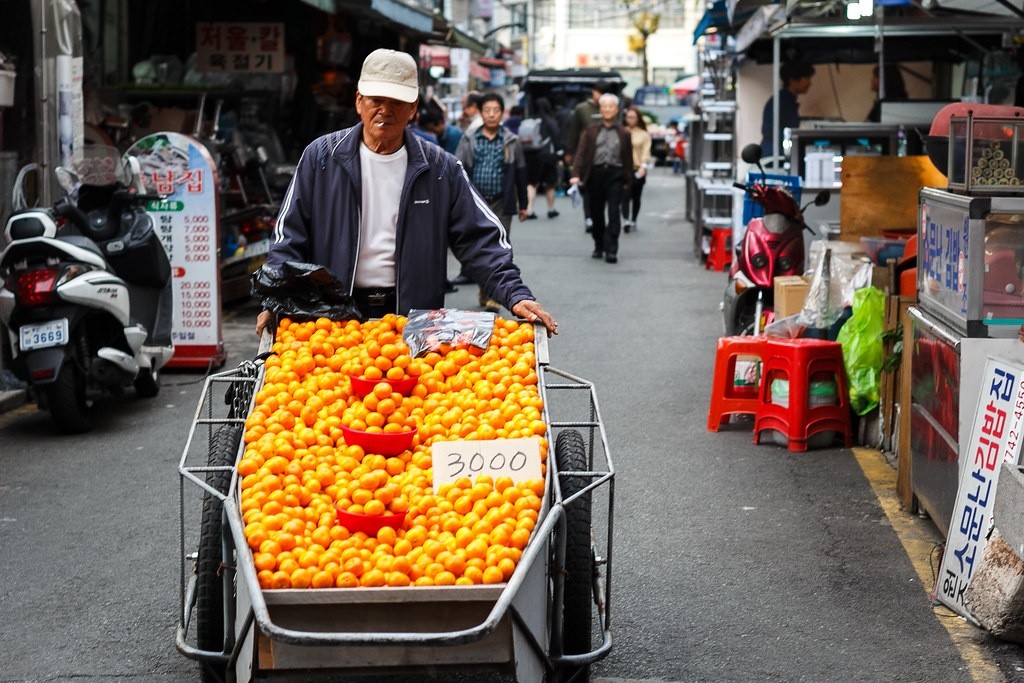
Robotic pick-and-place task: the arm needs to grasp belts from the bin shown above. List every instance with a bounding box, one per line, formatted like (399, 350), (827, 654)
(352, 292), (397, 307)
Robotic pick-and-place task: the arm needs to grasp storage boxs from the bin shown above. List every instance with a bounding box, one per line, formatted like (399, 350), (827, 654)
(140, 106), (197, 137)
(772, 276), (812, 328)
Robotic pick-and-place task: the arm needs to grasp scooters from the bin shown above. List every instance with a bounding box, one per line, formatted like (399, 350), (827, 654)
(723, 143), (831, 337)
(0, 144), (168, 432)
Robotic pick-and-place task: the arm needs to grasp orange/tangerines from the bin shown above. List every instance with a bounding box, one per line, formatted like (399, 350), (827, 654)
(238, 314), (548, 590)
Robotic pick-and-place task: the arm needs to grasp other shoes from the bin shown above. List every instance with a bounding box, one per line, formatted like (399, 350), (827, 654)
(623, 219), (637, 232)
(606, 251), (618, 263)
(527, 214), (538, 219)
(451, 274), (476, 285)
(592, 245), (604, 258)
(548, 210), (559, 217)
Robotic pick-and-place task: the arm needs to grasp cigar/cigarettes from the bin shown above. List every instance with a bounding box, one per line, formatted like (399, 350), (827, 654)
(377, 122), (384, 127)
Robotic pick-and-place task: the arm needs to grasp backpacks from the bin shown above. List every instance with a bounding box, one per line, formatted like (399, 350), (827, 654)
(517, 117), (553, 149)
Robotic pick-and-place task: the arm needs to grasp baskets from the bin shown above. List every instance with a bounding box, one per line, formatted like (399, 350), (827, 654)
(338, 420), (418, 459)
(347, 371), (419, 400)
(333, 496), (406, 537)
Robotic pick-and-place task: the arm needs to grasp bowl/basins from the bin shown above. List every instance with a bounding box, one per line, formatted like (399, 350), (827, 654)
(340, 424), (418, 459)
(346, 371), (418, 399)
(860, 238), (904, 267)
(333, 499), (410, 537)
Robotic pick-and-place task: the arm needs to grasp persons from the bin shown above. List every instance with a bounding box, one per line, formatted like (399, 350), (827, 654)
(454, 93), (529, 313)
(865, 64), (910, 122)
(503, 98), (577, 220)
(450, 94), (484, 286)
(760, 61), (817, 156)
(564, 80), (652, 264)
(417, 109), (463, 155)
(256, 48), (559, 340)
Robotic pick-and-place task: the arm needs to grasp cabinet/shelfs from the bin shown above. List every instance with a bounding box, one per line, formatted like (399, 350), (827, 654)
(686, 20), (1023, 278)
(896, 111), (1023, 543)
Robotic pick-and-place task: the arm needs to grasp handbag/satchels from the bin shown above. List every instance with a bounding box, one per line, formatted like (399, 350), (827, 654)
(836, 285), (886, 414)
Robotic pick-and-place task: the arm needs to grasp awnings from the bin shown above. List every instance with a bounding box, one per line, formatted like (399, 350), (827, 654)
(373, 0), (490, 56)
(693, 0), (757, 43)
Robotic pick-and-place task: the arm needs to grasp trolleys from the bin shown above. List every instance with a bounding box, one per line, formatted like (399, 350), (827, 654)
(176, 318), (615, 683)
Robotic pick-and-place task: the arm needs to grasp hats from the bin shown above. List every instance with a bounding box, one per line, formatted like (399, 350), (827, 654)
(357, 49), (419, 104)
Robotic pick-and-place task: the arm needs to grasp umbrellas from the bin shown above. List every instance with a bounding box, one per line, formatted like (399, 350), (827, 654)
(667, 75), (701, 99)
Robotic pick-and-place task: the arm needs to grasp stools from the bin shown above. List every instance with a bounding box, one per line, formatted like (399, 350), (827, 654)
(709, 336), (853, 452)
(705, 227), (733, 271)
